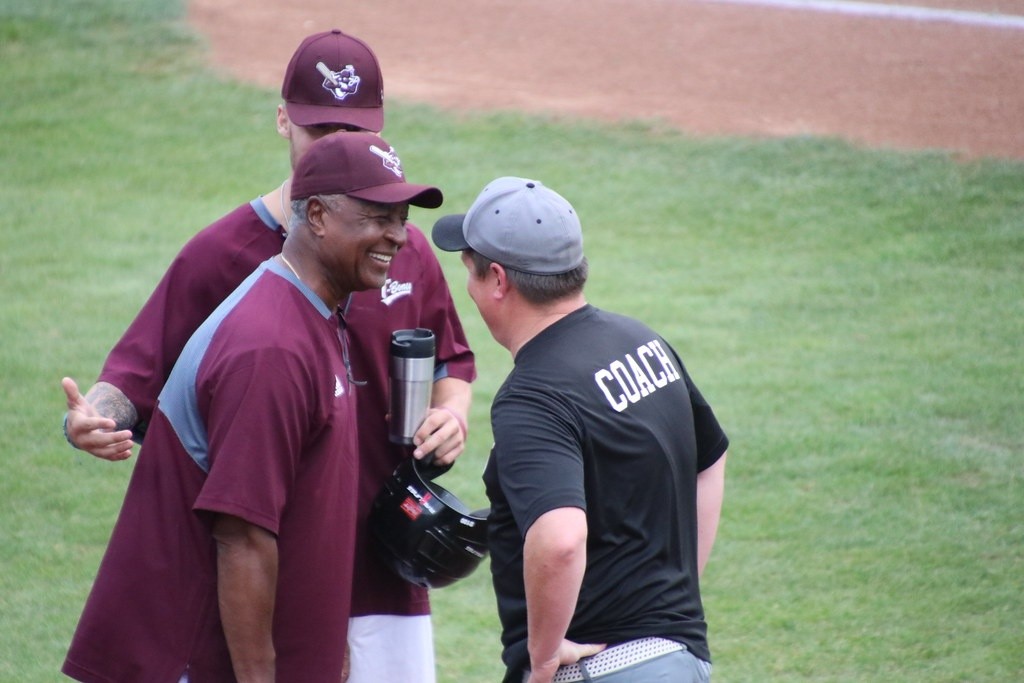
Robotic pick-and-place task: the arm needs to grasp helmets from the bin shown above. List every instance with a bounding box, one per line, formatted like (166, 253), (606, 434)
(366, 449), (491, 590)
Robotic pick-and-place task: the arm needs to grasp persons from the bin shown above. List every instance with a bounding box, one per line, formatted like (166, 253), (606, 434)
(431, 177), (731, 683)
(63, 26), (477, 683)
(63, 133), (445, 683)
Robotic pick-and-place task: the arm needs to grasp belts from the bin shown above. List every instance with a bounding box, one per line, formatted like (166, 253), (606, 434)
(524, 636), (688, 683)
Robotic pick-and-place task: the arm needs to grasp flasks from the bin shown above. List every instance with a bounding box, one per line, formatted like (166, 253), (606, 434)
(390, 326), (436, 445)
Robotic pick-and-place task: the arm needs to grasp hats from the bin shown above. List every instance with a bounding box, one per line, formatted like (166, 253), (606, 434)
(432, 176), (584, 275)
(289, 128), (443, 209)
(281, 28), (385, 132)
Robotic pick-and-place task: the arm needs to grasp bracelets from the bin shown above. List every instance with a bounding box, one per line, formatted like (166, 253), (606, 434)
(62, 414), (80, 450)
(281, 252), (303, 283)
(280, 179), (289, 225)
(437, 404), (468, 439)
